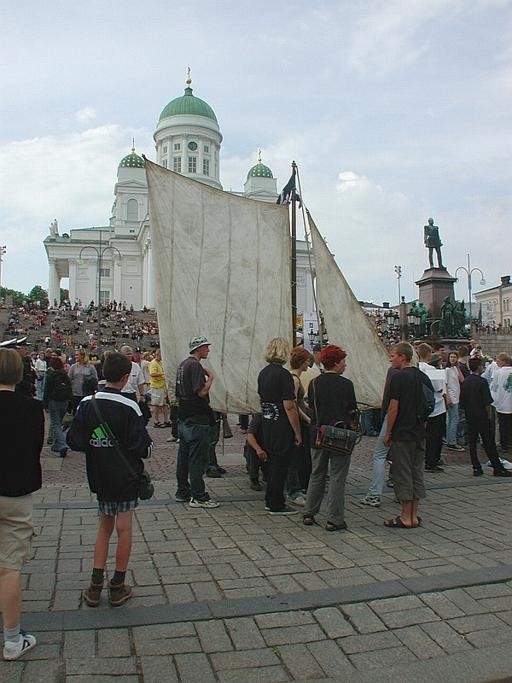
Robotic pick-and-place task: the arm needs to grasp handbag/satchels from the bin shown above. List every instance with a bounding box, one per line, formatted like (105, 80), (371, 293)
(312, 377), (358, 456)
(92, 394), (154, 501)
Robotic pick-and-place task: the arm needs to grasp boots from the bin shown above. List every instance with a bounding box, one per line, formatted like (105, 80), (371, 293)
(82, 583), (104, 606)
(108, 583), (133, 606)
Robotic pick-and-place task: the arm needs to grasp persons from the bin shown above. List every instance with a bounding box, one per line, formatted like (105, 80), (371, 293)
(424, 217), (445, 268)
(416, 342), (446, 473)
(40, 298), (159, 350)
(48, 218), (58, 234)
(30, 352), (37, 370)
(470, 346), (482, 357)
(170, 335), (221, 509)
(303, 345), (363, 531)
(66, 353), (155, 609)
(88, 347), (101, 368)
(141, 351), (151, 391)
(458, 346), (470, 375)
(36, 353), (40, 360)
(359, 343), (418, 508)
(206, 412), (226, 478)
(427, 353), (443, 369)
(444, 350), (465, 452)
(258, 337), (302, 515)
(489, 353), (512, 452)
(441, 296), (454, 339)
(67, 349), (98, 416)
(0, 348), (45, 661)
(148, 348), (176, 429)
(467, 339), (477, 355)
(47, 350), (64, 374)
(34, 352), (47, 399)
(454, 299), (465, 337)
(417, 302), (428, 333)
(410, 302), (418, 317)
(382, 341), (436, 528)
(459, 357), (512, 478)
(288, 348), (311, 506)
(15, 337), (39, 398)
(43, 357), (71, 458)
(243, 412), (267, 492)
(1, 295), (40, 337)
(433, 344), (446, 353)
(120, 346), (145, 404)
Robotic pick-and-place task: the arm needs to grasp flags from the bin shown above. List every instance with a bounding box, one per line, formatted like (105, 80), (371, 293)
(276, 160), (303, 207)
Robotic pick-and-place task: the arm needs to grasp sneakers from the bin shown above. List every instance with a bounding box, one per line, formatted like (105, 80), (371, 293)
(268, 505), (299, 515)
(446, 444), (465, 452)
(473, 468), (483, 476)
(2, 635), (36, 661)
(287, 495), (305, 507)
(360, 495), (381, 507)
(218, 468), (226, 473)
(206, 469), (221, 478)
(493, 468), (512, 477)
(188, 496), (220, 509)
(265, 504), (270, 511)
(175, 489), (191, 502)
(165, 437), (175, 442)
(250, 479), (261, 491)
(423, 466), (444, 473)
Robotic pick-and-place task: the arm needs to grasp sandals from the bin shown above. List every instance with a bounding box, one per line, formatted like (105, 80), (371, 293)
(163, 421), (173, 427)
(325, 521), (346, 531)
(302, 514), (314, 525)
(153, 421), (165, 428)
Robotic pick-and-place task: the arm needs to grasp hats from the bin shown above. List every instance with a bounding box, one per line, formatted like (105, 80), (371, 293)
(0, 338), (21, 348)
(429, 353), (442, 363)
(188, 334), (212, 354)
(17, 336), (32, 346)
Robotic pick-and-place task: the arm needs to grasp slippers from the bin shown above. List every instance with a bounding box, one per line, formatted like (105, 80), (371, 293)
(383, 516), (416, 529)
